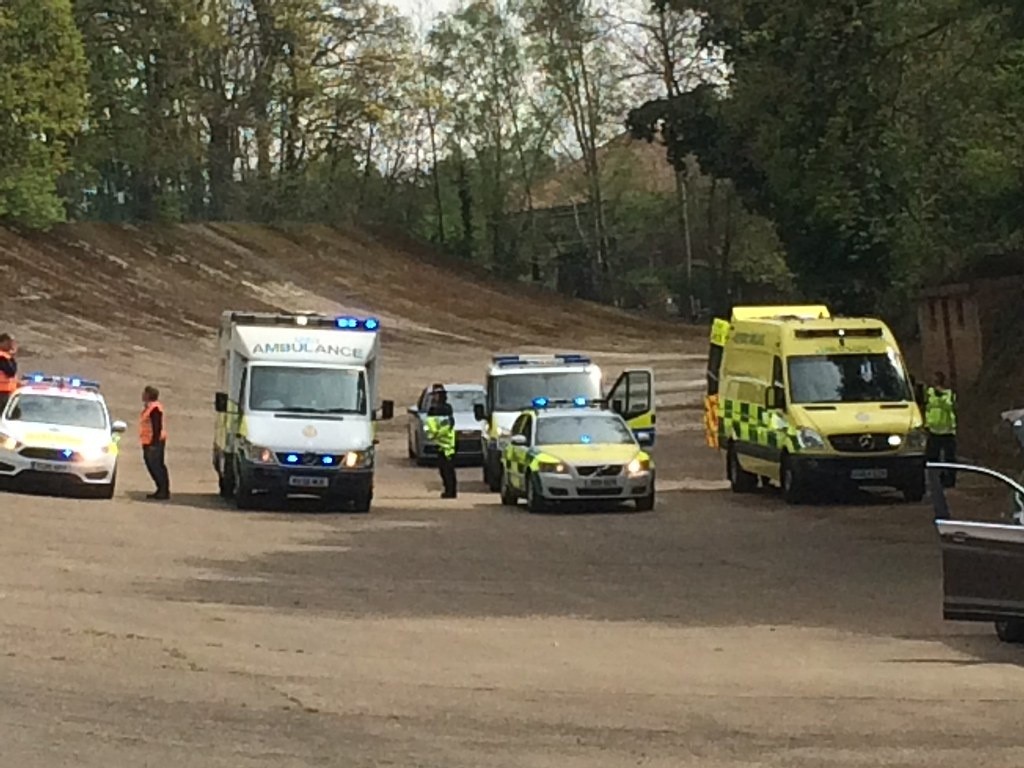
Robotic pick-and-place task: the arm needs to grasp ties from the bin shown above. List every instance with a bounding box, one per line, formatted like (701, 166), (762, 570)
(864, 363), (867, 373)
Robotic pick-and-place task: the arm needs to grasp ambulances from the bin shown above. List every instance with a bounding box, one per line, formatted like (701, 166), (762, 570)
(498, 402), (656, 511)
(0, 373), (128, 499)
(213, 311), (394, 512)
(702, 304), (928, 503)
(475, 354), (656, 496)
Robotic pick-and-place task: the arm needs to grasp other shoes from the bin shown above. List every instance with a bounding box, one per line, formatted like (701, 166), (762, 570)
(442, 493), (456, 498)
(146, 491), (170, 499)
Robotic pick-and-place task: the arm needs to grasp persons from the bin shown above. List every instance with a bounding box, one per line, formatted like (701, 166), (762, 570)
(0, 332), (19, 419)
(916, 371), (957, 487)
(139, 386), (170, 500)
(425, 404), (458, 498)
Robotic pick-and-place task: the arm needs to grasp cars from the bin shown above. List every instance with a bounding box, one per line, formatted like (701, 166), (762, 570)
(924, 410), (1024, 642)
(407, 382), (489, 467)
